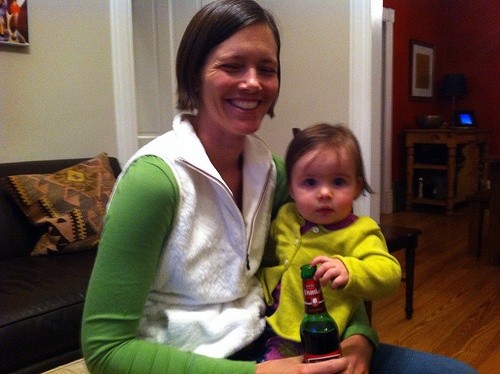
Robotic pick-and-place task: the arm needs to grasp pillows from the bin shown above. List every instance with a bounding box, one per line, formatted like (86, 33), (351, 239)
(2, 151), (114, 256)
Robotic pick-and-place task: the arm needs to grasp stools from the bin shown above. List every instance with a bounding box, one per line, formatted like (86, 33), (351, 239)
(362, 224), (421, 327)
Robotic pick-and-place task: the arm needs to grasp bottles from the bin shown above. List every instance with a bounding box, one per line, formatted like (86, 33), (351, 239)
(299, 265), (341, 364)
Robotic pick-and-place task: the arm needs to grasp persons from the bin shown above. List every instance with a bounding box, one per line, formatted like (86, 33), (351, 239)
(254, 122), (401, 363)
(80, 0), (482, 374)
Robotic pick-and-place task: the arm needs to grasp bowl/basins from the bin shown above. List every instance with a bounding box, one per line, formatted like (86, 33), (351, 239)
(414, 115), (444, 128)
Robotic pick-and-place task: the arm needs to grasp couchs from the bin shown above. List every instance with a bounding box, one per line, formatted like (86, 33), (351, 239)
(0, 156), (122, 374)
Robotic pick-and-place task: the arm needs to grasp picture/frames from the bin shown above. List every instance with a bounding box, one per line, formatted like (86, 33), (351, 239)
(408, 40), (436, 101)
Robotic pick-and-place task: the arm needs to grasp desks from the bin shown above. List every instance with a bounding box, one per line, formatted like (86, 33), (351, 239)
(405, 127), (487, 213)
